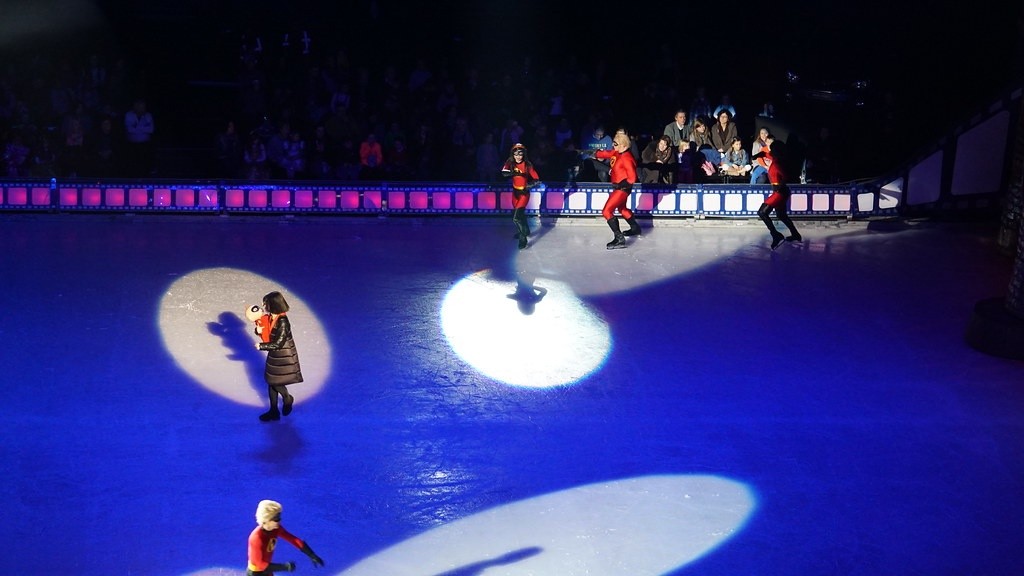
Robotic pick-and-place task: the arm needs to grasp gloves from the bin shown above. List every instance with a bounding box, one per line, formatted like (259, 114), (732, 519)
(576, 148), (598, 161)
(263, 561), (296, 573)
(527, 178), (538, 184)
(612, 178), (632, 190)
(751, 151), (766, 160)
(300, 541), (325, 569)
(775, 175), (787, 193)
(501, 170), (516, 178)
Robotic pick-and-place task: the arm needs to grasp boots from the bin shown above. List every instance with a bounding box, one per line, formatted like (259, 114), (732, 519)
(514, 220), (528, 252)
(775, 201), (803, 244)
(606, 217), (627, 249)
(622, 214), (642, 238)
(260, 409), (281, 422)
(514, 218), (530, 239)
(756, 203), (785, 251)
(281, 395), (293, 416)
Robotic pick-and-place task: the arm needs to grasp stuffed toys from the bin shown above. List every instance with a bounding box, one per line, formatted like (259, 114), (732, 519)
(245, 305), (271, 343)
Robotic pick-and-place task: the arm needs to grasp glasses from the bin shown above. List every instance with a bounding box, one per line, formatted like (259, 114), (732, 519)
(274, 516), (282, 523)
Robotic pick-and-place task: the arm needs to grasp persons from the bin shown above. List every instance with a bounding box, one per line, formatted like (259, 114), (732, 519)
(247, 500), (326, 575)
(753, 140), (803, 249)
(209, 106), (778, 185)
(576, 129), (642, 249)
(255, 291), (304, 420)
(501, 143), (540, 249)
(0, 92), (156, 179)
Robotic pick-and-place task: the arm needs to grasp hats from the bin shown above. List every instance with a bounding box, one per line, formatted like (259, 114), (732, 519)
(512, 143), (525, 149)
